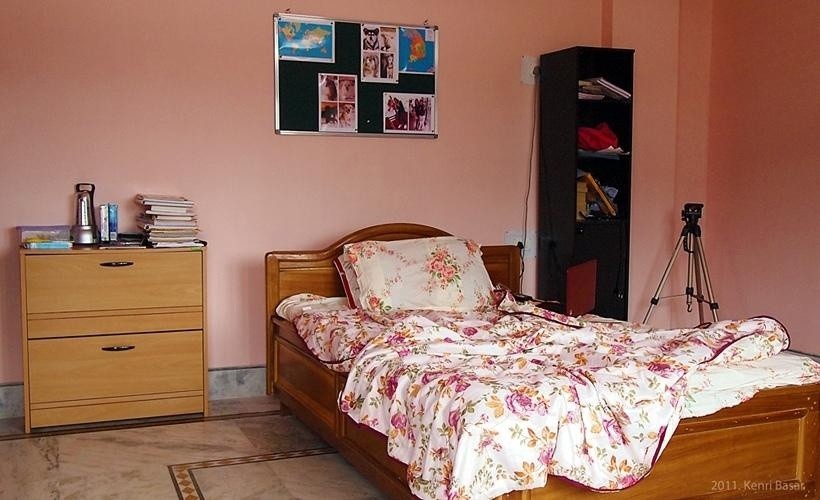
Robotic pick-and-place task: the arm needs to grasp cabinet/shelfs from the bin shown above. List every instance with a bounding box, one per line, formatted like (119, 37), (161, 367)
(19, 242), (208, 432)
(538, 46), (635, 320)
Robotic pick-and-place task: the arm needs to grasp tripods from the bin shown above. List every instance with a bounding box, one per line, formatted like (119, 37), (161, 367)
(643, 203), (718, 325)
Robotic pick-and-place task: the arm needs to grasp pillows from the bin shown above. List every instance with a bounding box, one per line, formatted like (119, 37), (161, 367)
(333, 253), (363, 309)
(342, 237), (495, 313)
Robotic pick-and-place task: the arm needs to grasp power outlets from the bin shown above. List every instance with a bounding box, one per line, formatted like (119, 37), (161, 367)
(521, 54), (541, 84)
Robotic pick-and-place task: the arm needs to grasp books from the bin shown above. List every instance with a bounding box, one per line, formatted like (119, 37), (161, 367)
(585, 172), (616, 216)
(577, 76), (632, 101)
(117, 194), (208, 248)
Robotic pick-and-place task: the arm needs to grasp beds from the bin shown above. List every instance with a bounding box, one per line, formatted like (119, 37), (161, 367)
(265, 223), (820, 500)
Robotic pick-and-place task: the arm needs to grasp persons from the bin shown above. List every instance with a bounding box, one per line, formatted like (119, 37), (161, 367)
(385, 95), (433, 131)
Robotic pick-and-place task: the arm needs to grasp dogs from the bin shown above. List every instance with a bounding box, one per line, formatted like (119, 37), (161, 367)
(339, 104), (354, 127)
(383, 54), (394, 79)
(380, 32), (395, 51)
(340, 80), (353, 99)
(363, 28), (379, 50)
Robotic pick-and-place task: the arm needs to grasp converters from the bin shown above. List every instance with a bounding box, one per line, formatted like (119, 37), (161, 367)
(514, 293), (532, 301)
